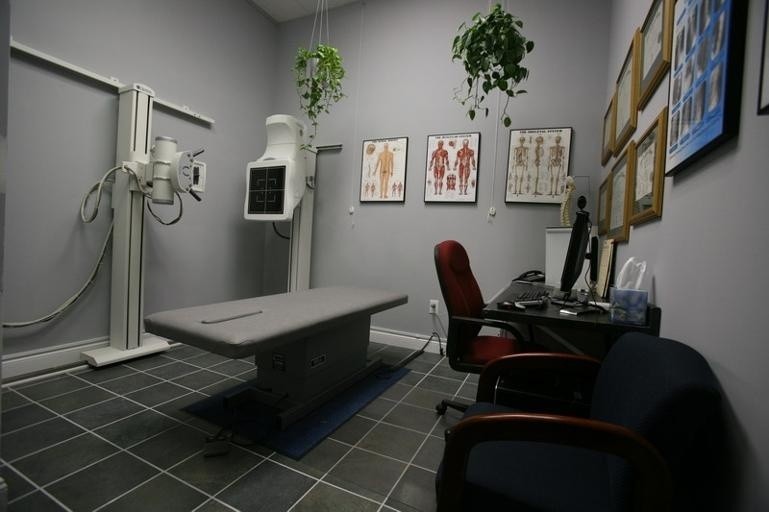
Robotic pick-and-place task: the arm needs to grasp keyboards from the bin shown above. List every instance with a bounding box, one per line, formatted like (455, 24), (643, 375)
(514, 289), (549, 310)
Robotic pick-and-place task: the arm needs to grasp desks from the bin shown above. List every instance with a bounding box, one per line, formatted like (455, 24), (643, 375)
(483, 280), (660, 354)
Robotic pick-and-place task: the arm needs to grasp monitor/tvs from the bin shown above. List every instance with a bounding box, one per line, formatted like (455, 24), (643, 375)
(551, 212), (589, 302)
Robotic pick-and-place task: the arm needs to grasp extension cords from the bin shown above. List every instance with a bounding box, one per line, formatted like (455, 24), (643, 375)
(590, 236), (598, 281)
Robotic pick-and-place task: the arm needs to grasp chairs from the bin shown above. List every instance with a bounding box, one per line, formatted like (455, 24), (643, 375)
(435, 240), (546, 414)
(436, 332), (721, 511)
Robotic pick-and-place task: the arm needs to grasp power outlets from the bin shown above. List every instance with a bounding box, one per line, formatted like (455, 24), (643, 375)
(428, 299), (439, 314)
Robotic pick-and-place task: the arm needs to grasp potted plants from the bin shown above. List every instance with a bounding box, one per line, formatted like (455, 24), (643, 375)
(293, 44), (345, 149)
(451, 3), (534, 127)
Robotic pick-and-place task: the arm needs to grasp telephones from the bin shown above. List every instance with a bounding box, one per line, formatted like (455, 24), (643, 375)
(511, 269), (545, 282)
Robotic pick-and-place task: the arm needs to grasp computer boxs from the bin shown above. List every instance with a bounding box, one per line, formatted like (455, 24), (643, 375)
(544, 226), (599, 292)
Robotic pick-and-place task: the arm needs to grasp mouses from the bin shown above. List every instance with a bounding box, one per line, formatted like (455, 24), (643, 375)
(499, 300), (513, 309)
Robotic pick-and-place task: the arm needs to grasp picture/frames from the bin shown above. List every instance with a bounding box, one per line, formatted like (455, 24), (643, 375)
(360, 137), (408, 202)
(424, 132), (480, 203)
(757, 0), (769, 114)
(505, 127), (572, 204)
(596, 1), (674, 245)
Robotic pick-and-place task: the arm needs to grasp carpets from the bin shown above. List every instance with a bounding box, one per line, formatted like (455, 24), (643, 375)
(178, 360), (413, 461)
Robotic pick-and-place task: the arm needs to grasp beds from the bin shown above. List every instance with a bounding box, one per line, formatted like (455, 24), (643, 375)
(143, 284), (408, 432)
